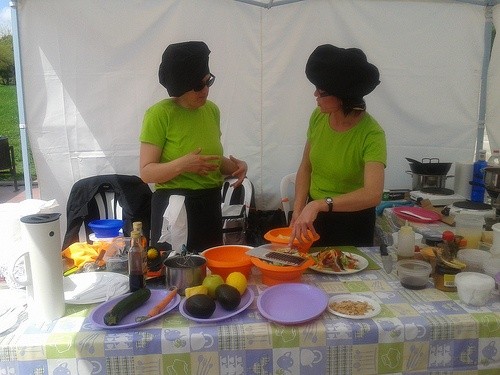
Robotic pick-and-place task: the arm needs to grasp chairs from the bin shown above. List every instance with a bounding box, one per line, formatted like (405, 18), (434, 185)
(77, 172), (297, 244)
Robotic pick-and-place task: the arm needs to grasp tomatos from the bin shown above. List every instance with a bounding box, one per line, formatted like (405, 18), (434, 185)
(442, 230), (455, 240)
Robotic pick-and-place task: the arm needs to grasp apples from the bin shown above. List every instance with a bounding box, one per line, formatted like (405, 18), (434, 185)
(203, 275), (224, 297)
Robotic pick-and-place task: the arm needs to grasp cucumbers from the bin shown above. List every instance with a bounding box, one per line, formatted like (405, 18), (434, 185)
(104, 287), (151, 325)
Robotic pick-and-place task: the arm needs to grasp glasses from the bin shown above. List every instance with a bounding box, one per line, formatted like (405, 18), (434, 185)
(193, 74), (215, 92)
(316, 85), (332, 98)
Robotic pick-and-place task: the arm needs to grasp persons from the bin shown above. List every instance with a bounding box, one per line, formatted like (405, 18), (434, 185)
(139, 40), (248, 252)
(287, 44), (388, 248)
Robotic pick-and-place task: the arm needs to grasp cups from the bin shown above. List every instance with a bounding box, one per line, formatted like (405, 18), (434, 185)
(453, 213), (486, 249)
(162, 254), (207, 296)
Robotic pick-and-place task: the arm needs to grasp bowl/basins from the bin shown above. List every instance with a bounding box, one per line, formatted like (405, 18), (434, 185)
(392, 232), (423, 248)
(422, 247), (442, 264)
(199, 228), (320, 285)
(88, 219), (126, 238)
(393, 243), (429, 261)
(396, 259), (433, 290)
(455, 223), (500, 307)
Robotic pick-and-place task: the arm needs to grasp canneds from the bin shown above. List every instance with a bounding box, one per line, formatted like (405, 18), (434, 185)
(434, 264), (460, 292)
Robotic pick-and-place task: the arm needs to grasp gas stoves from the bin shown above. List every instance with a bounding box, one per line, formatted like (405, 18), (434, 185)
(441, 201), (496, 220)
(409, 187), (467, 206)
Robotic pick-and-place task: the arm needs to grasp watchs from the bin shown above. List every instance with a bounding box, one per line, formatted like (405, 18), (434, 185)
(325, 197), (333, 212)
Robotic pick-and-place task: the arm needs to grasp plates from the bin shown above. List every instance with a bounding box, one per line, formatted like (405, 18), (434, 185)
(63, 250), (382, 329)
(391, 206), (441, 223)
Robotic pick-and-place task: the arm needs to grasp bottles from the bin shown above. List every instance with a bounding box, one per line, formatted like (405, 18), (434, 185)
(131, 222), (147, 275)
(129, 231), (146, 292)
(471, 147), (500, 205)
(433, 230), (467, 292)
(397, 220), (415, 275)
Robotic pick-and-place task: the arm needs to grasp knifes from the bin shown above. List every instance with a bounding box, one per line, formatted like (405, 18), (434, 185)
(380, 244), (393, 274)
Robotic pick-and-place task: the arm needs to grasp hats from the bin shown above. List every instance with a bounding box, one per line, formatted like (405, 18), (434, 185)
(158, 40), (211, 98)
(306, 43), (380, 99)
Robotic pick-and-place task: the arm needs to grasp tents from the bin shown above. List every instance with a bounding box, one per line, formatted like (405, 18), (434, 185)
(10, 0), (499, 206)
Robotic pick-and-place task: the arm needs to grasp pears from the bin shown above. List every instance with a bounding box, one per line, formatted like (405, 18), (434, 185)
(185, 285), (207, 298)
(226, 272), (247, 295)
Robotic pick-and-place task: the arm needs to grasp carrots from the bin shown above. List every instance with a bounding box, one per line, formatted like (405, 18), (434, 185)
(148, 288), (178, 317)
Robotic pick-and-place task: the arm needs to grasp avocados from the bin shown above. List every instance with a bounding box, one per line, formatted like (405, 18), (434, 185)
(214, 284), (242, 311)
(186, 294), (215, 320)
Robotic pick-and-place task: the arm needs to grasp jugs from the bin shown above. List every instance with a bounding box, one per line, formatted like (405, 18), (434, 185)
(6, 211), (65, 322)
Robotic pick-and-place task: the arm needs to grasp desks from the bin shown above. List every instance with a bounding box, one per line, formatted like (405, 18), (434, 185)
(381, 197), (461, 241)
(0, 245), (500, 375)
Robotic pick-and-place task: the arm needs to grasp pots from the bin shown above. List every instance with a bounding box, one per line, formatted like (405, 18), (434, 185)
(404, 157), (453, 176)
(405, 171), (455, 191)
